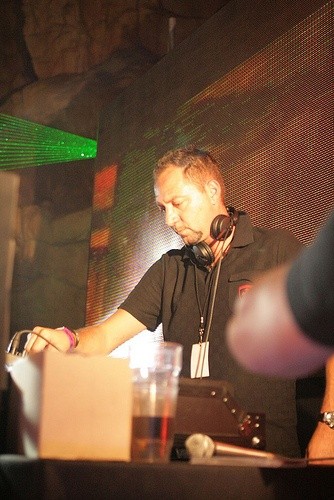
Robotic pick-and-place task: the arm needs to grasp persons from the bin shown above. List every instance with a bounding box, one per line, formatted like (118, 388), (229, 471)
(224, 212), (334, 381)
(25, 149), (334, 465)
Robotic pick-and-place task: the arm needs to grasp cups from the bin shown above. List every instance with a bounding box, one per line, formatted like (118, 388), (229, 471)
(131, 342), (183, 462)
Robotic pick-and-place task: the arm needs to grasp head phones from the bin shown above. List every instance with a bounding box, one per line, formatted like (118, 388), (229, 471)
(192, 206), (239, 266)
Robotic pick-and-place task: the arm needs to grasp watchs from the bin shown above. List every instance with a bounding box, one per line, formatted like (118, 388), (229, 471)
(319, 411), (334, 429)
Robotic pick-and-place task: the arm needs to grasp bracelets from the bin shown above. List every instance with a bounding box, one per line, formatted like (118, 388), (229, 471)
(55, 327), (80, 353)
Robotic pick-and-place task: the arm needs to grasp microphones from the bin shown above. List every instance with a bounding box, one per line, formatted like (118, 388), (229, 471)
(183, 432), (298, 465)
